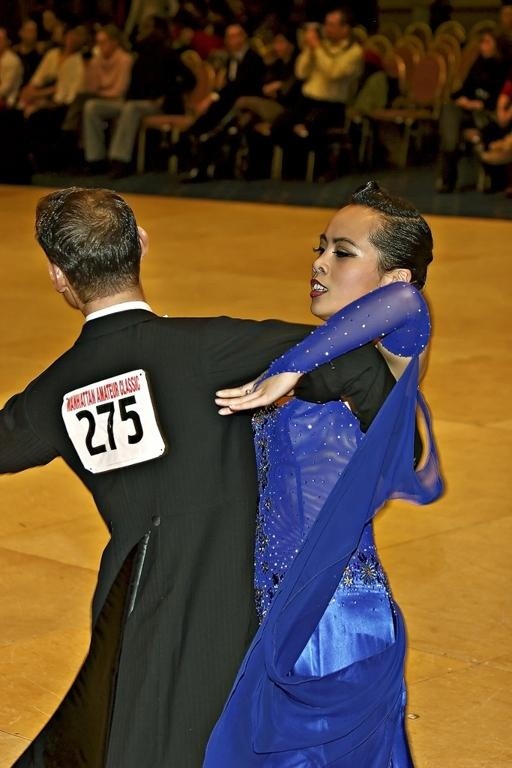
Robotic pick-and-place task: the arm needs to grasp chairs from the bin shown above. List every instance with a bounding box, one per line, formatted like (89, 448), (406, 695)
(137, 19), (501, 201)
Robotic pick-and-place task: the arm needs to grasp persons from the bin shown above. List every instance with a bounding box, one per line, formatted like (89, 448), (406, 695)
(0, 181), (432, 768)
(194, 187), (449, 767)
(2, 1), (386, 186)
(429, 2), (453, 33)
(440, 1), (511, 195)
